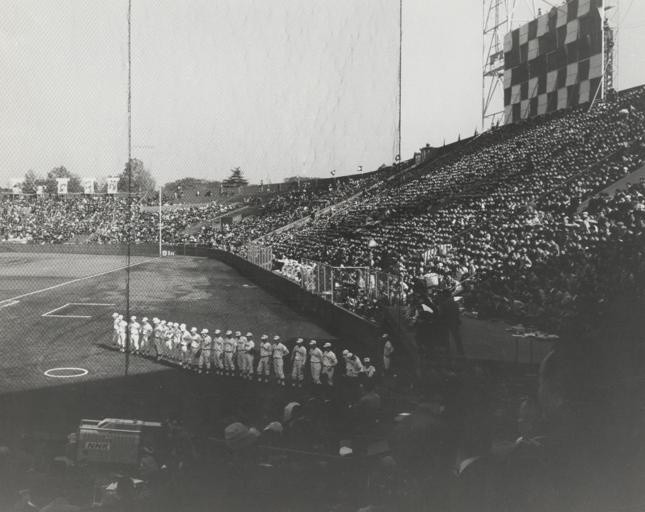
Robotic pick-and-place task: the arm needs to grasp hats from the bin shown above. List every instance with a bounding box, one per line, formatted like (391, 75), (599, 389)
(214, 329), (222, 335)
(223, 421), (257, 451)
(263, 420), (284, 434)
(260, 334), (268, 340)
(341, 349), (355, 358)
(381, 333), (390, 339)
(309, 339), (317, 346)
(295, 337), (305, 344)
(112, 312), (187, 328)
(225, 329), (232, 336)
(190, 326), (198, 332)
(272, 334), (280, 341)
(235, 331), (241, 337)
(200, 328), (210, 334)
(283, 402), (301, 422)
(246, 332), (254, 337)
(322, 341), (333, 348)
(364, 356), (371, 364)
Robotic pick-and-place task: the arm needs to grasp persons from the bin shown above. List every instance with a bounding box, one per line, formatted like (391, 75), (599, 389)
(2, 312), (645, 512)
(2, 82), (645, 326)
